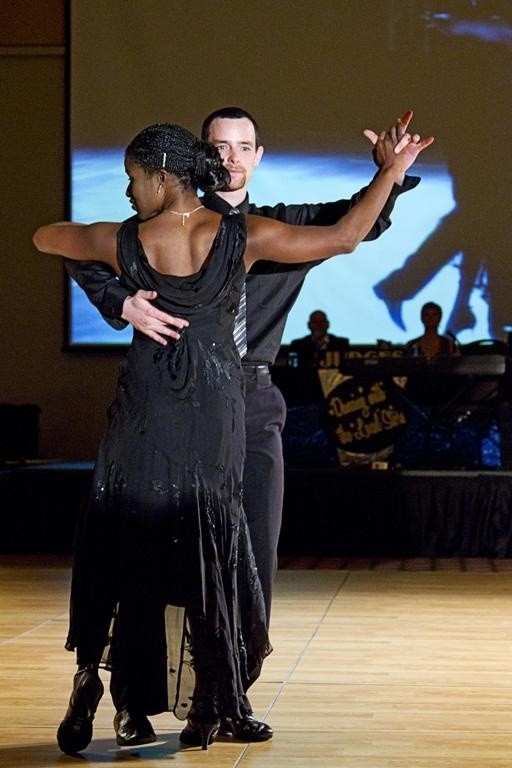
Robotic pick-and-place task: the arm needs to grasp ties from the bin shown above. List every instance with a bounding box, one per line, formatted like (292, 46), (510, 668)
(229, 208), (250, 362)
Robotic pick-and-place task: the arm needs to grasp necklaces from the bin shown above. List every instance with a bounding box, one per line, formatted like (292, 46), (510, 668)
(163, 205), (204, 228)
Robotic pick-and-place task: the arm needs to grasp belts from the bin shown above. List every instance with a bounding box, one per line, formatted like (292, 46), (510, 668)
(242, 362), (270, 375)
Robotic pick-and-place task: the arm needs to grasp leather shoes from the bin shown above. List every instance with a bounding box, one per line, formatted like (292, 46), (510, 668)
(222, 714), (276, 744)
(110, 711), (158, 746)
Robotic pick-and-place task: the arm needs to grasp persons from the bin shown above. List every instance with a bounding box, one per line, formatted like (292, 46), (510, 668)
(406, 302), (458, 359)
(371, 153), (492, 335)
(287, 310), (353, 355)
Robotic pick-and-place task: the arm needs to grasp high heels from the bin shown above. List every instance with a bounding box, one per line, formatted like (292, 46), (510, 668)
(58, 663), (104, 754)
(191, 707), (219, 748)
